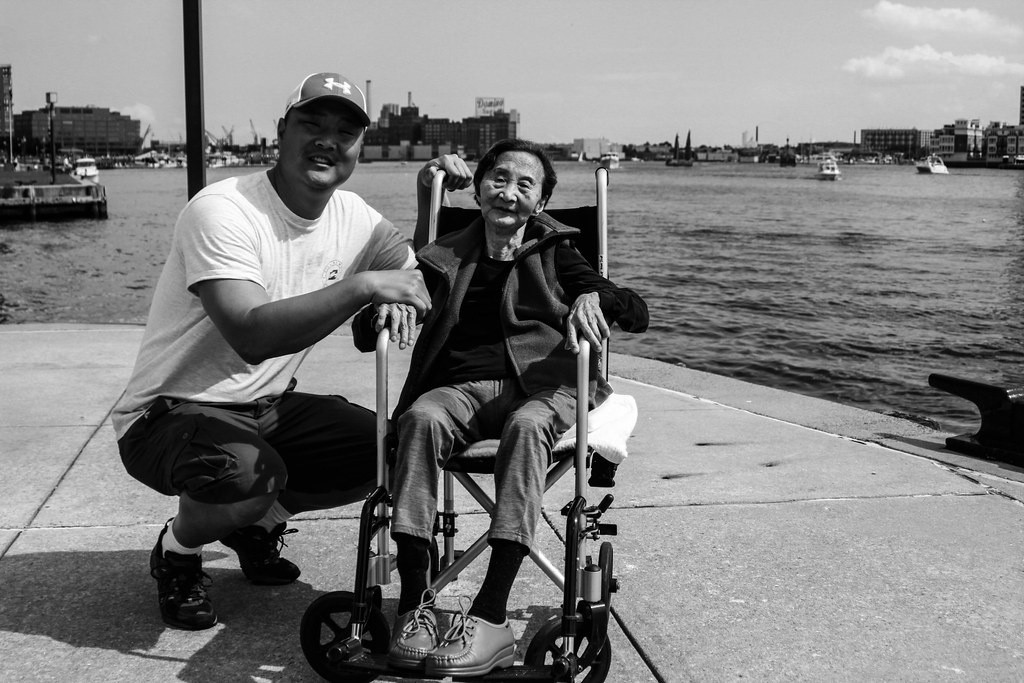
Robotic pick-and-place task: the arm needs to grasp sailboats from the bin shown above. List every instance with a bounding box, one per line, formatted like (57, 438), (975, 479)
(665, 128), (692, 167)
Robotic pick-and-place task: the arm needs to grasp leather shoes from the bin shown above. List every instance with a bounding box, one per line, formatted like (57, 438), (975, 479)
(386, 588), (518, 677)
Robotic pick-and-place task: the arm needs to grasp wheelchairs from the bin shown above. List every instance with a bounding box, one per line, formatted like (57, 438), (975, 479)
(300, 167), (623, 683)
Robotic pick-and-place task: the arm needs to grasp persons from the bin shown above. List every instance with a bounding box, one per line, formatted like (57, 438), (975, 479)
(111, 71), (474, 630)
(351, 139), (648, 676)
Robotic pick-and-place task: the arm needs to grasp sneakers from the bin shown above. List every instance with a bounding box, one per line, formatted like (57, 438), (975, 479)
(150, 517), (219, 631)
(218, 520), (301, 587)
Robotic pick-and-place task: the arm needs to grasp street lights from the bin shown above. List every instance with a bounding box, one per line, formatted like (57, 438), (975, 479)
(46, 91), (58, 183)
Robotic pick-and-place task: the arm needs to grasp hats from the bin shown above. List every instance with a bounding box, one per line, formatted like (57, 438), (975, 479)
(284, 71), (371, 132)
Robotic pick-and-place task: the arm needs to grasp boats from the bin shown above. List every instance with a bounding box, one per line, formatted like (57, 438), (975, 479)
(915, 152), (950, 174)
(600, 152), (619, 169)
(779, 137), (797, 167)
(814, 158), (842, 180)
(75, 155), (99, 176)
